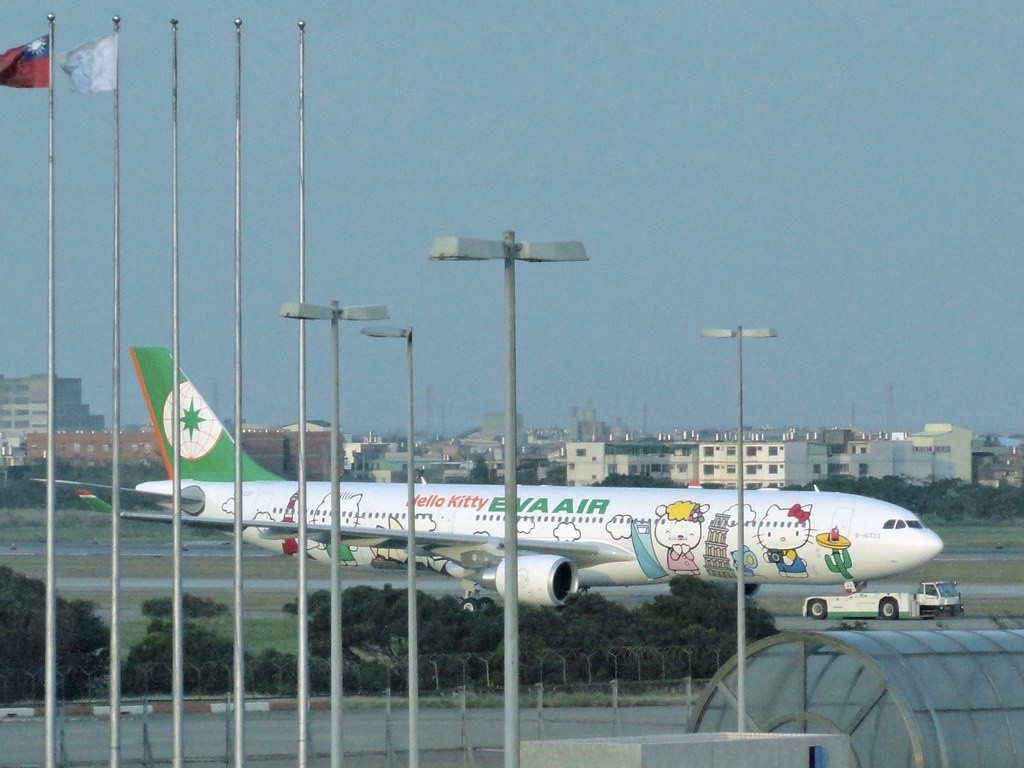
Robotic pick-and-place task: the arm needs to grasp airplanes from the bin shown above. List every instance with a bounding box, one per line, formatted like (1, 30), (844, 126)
(31, 348), (946, 610)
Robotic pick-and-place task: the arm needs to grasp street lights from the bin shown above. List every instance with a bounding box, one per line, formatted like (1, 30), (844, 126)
(359, 325), (421, 768)
(699, 328), (779, 734)
(280, 298), (386, 768)
(428, 231), (589, 768)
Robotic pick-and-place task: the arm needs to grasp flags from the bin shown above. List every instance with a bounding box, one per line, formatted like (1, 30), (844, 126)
(0, 32), (50, 88)
(53, 32), (118, 93)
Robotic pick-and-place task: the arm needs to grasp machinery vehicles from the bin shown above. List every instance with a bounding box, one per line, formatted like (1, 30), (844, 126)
(802, 582), (963, 620)
